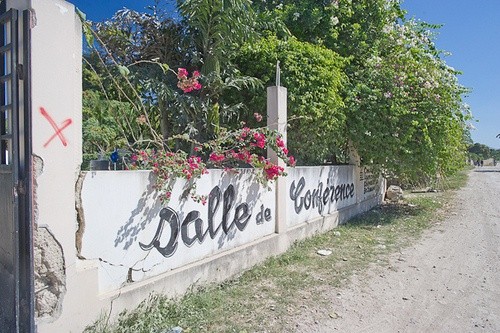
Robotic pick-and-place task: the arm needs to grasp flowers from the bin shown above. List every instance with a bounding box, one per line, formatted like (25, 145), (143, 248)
(120, 124), (292, 211)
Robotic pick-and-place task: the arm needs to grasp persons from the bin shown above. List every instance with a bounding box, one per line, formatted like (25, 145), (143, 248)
(477, 156), (497, 165)
